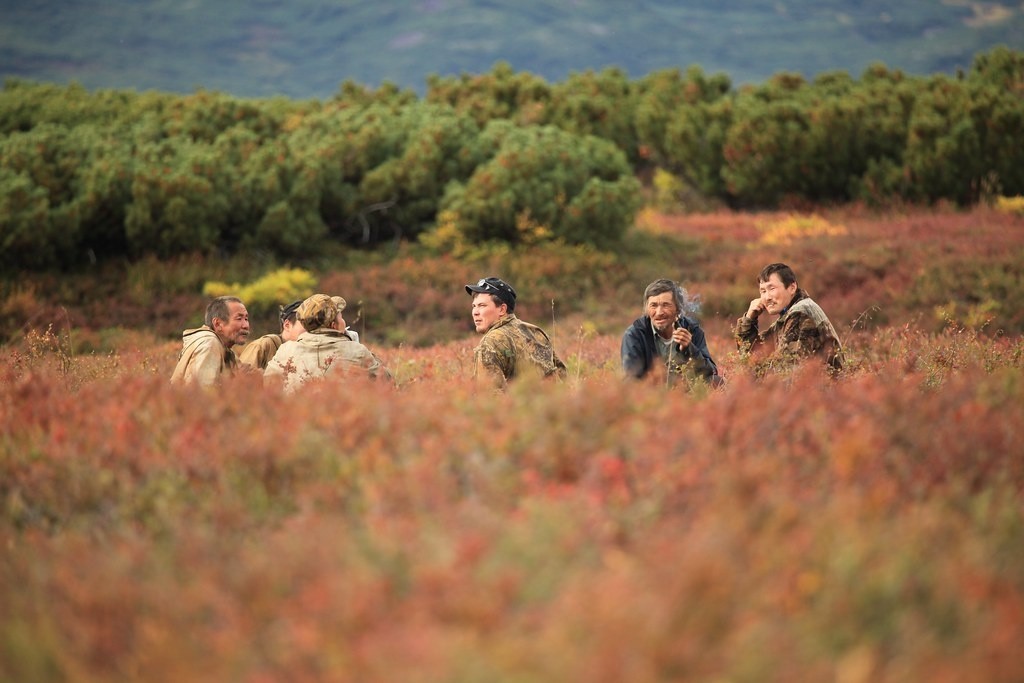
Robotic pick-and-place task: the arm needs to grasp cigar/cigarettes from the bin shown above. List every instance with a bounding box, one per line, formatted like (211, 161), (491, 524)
(346, 326), (350, 329)
(676, 329), (680, 335)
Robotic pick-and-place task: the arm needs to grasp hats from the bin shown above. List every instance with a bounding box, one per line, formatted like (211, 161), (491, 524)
(280, 300), (303, 323)
(465, 277), (516, 309)
(296, 294), (347, 332)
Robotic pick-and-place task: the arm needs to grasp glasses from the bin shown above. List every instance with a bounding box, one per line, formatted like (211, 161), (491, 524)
(477, 279), (500, 291)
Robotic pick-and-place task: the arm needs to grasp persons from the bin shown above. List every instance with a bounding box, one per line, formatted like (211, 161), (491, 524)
(619, 277), (721, 391)
(735, 263), (845, 371)
(170, 296), (309, 381)
(264, 294), (390, 390)
(465, 278), (567, 385)
